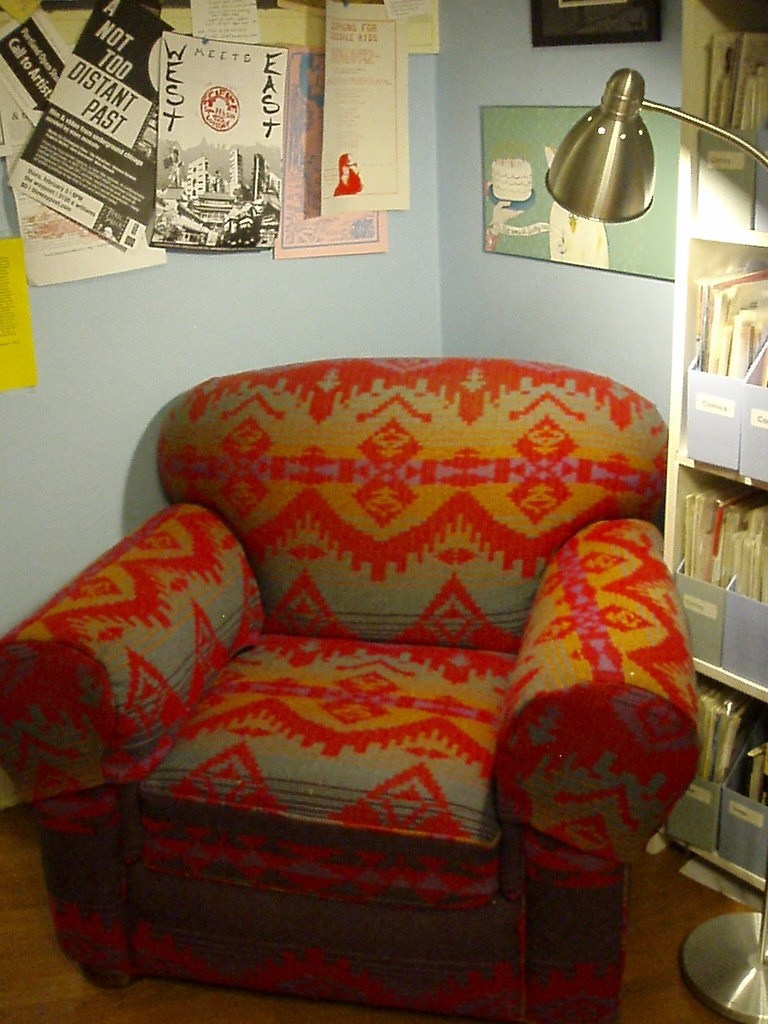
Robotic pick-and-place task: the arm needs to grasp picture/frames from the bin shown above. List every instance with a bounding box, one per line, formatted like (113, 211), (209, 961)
(529, 0), (662, 48)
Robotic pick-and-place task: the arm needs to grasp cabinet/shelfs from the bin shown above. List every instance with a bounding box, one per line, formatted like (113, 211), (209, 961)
(665, 0), (768, 895)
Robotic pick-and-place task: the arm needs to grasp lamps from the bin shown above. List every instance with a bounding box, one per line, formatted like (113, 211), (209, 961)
(543, 66), (768, 1024)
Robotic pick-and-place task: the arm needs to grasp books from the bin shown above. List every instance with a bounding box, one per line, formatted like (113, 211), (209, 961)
(707, 32), (768, 130)
(696, 269), (768, 388)
(684, 492), (768, 604)
(698, 686), (768, 807)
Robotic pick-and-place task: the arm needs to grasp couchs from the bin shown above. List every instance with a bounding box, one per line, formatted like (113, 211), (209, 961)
(0, 354), (705, 1022)
